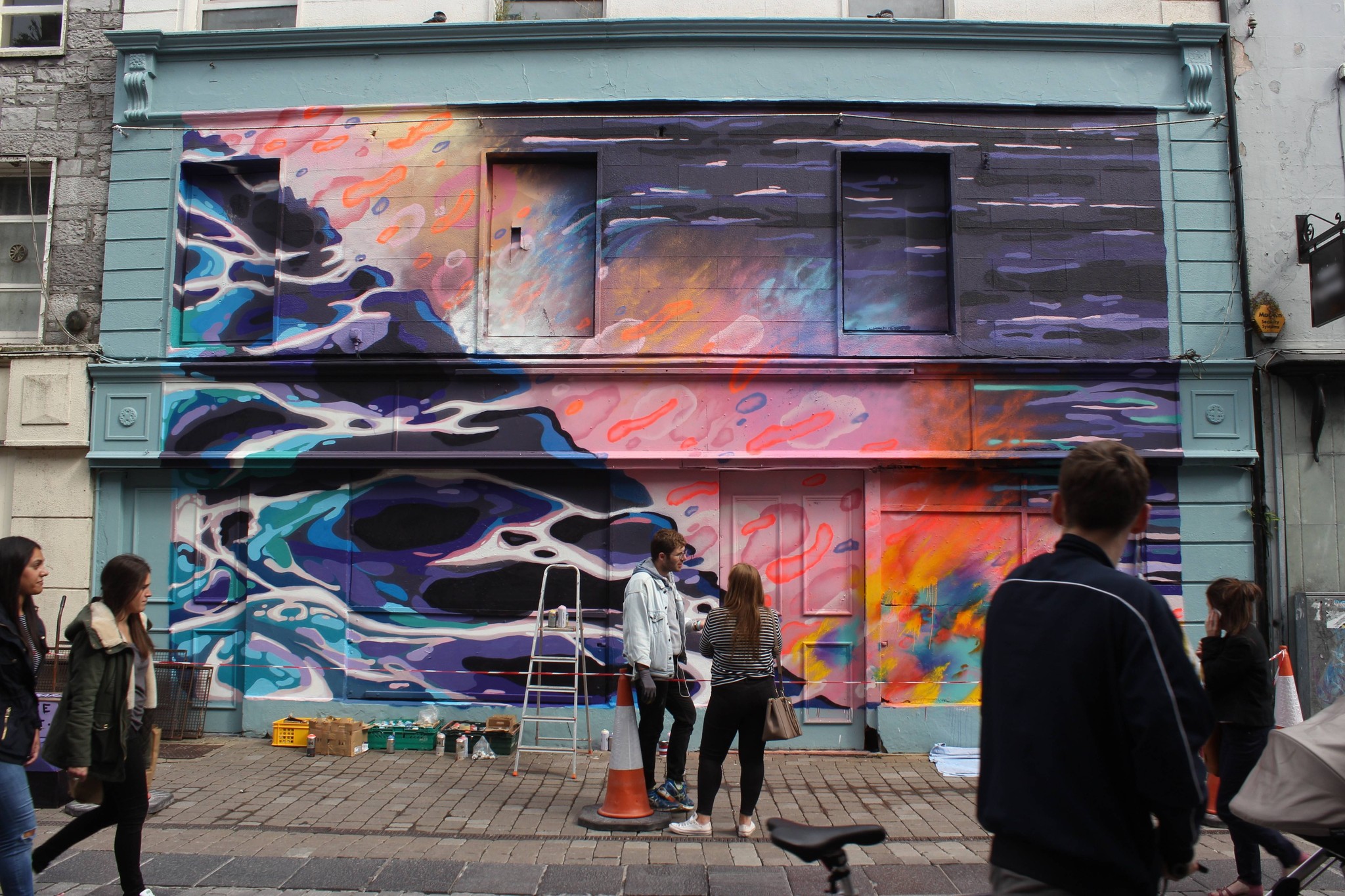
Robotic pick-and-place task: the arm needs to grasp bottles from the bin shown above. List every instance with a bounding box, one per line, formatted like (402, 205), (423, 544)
(609, 732), (613, 751)
(306, 734), (316, 757)
(600, 729), (609, 751)
(374, 720), (419, 730)
(386, 735), (396, 754)
(548, 609), (557, 628)
(658, 740), (669, 757)
(456, 733), (468, 760)
(436, 733), (446, 756)
(446, 722), (484, 731)
(558, 605), (569, 628)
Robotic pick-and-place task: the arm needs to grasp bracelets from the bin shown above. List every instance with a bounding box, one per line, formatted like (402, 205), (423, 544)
(698, 620), (701, 629)
(694, 621), (700, 631)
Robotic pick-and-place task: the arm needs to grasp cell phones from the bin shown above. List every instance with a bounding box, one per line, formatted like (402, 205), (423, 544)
(1212, 608), (1222, 619)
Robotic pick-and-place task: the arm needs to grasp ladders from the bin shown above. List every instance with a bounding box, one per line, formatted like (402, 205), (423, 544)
(511, 564), (592, 778)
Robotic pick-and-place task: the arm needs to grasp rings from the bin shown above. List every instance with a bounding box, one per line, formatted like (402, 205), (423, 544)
(74, 778), (80, 781)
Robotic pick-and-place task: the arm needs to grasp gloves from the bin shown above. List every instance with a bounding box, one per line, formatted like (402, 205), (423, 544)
(637, 669), (656, 705)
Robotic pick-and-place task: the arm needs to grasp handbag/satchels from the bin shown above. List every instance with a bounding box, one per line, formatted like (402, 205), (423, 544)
(69, 726), (161, 807)
(762, 697), (804, 741)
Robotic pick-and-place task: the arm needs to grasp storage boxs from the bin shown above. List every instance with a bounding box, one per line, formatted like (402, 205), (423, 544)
(364, 719), (444, 751)
(307, 718), (375, 757)
(485, 715), (516, 733)
(440, 720), (520, 756)
(271, 717), (353, 747)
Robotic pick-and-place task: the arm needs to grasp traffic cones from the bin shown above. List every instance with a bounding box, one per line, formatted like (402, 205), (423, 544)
(1200, 740), (1229, 829)
(1275, 645), (1304, 729)
(577, 667), (674, 833)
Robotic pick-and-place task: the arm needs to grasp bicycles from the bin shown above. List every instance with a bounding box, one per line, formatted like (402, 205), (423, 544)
(763, 806), (1344, 896)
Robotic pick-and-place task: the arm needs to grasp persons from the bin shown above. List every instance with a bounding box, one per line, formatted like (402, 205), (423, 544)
(32, 554), (158, 896)
(977, 441), (1210, 896)
(623, 529), (706, 810)
(1194, 578), (1314, 896)
(0, 536), (49, 896)
(669, 563), (782, 837)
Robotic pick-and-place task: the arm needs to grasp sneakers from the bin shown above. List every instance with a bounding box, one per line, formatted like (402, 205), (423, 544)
(659, 780), (694, 811)
(738, 821), (756, 837)
(646, 788), (680, 812)
(668, 812), (712, 837)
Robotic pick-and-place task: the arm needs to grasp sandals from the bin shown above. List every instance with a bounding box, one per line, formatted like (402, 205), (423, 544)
(1282, 852), (1311, 877)
(1208, 875), (1265, 896)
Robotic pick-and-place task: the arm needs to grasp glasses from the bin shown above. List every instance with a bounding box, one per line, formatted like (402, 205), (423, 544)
(665, 549), (688, 559)
